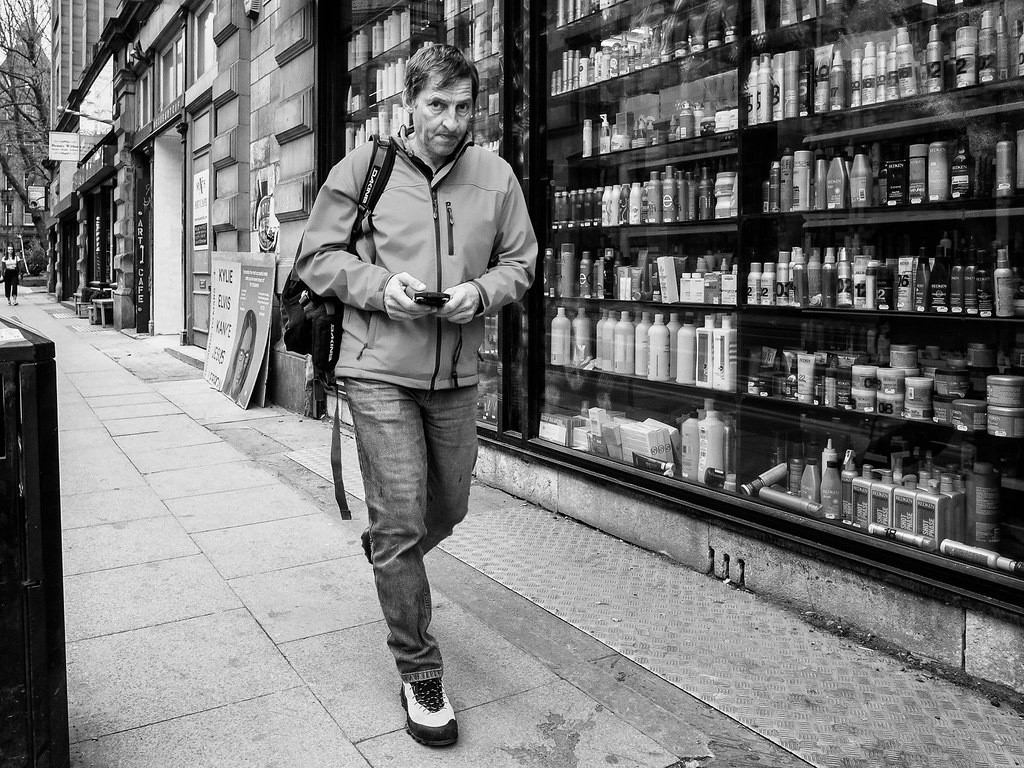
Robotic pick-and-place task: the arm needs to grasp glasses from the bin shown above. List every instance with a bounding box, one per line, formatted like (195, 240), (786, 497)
(237, 349), (250, 364)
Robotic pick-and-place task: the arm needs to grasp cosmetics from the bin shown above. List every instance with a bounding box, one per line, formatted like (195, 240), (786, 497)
(345, 0), (1024, 578)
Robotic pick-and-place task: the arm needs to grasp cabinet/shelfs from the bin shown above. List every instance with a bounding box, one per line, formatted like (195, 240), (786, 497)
(313, 0), (1024, 626)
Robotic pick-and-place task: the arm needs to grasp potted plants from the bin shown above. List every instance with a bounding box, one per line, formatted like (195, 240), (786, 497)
(87, 290), (108, 325)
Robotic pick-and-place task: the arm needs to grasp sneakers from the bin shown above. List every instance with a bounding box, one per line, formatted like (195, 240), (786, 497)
(360, 527), (374, 565)
(399, 678), (458, 745)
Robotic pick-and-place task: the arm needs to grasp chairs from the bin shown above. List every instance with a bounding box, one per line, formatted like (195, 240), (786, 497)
(91, 290), (117, 328)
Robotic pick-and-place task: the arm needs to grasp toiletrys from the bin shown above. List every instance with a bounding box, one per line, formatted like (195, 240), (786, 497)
(346, 0), (1024, 572)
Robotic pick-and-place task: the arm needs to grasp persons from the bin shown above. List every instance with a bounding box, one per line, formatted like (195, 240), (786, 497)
(295, 43), (539, 748)
(0, 242), (23, 306)
(224, 310), (256, 400)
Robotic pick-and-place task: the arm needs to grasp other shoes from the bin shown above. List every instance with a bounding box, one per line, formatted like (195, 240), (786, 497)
(8, 301), (11, 305)
(13, 301), (18, 306)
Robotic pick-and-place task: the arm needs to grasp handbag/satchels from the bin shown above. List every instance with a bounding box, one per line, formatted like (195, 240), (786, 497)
(281, 133), (397, 370)
